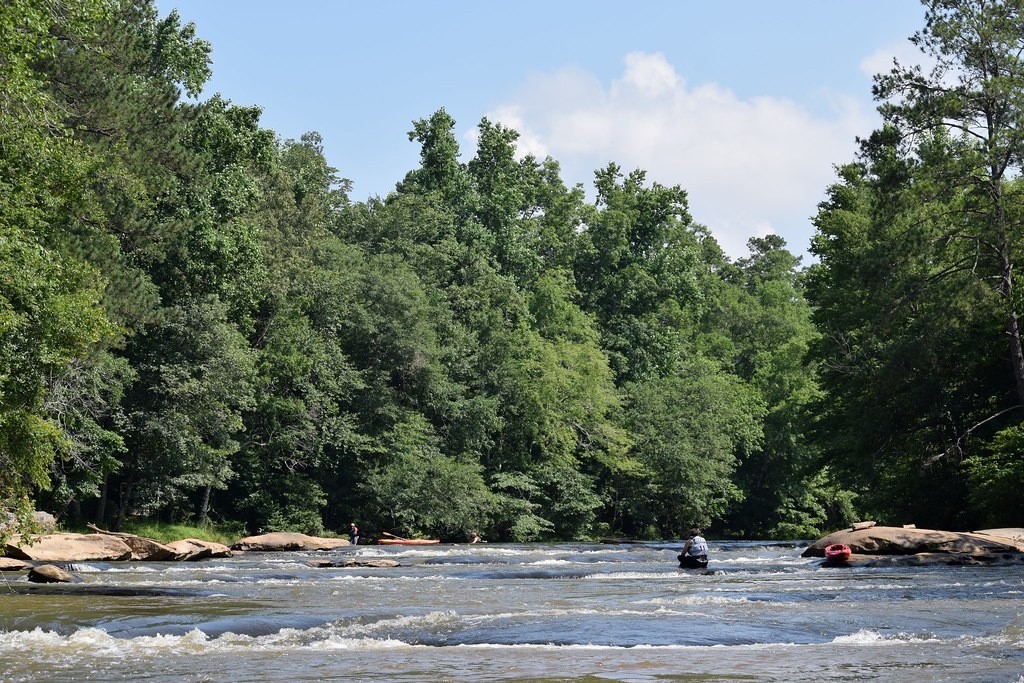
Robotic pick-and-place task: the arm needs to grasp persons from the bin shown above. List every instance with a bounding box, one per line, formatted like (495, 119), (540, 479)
(469, 532), (481, 544)
(351, 523), (359, 546)
(677, 529), (708, 569)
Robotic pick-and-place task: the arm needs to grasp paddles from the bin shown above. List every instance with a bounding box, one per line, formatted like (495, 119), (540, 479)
(383, 532), (406, 540)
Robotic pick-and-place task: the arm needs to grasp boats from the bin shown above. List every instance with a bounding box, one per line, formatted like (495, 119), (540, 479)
(824, 543), (851, 563)
(678, 553), (708, 569)
(376, 537), (441, 546)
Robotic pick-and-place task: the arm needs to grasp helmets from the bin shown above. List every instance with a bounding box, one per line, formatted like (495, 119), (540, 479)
(471, 532), (476, 537)
